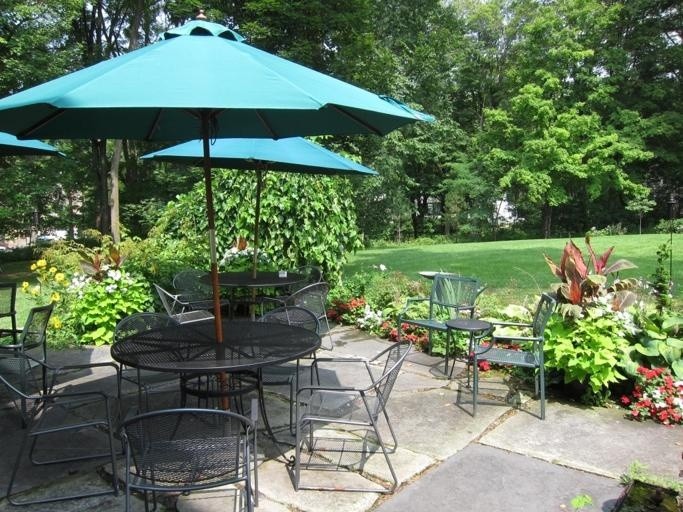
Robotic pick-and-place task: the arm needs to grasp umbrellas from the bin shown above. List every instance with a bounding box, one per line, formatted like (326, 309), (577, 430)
(1, 132), (67, 161)
(135, 134), (381, 321)
(0, 13), (439, 439)
